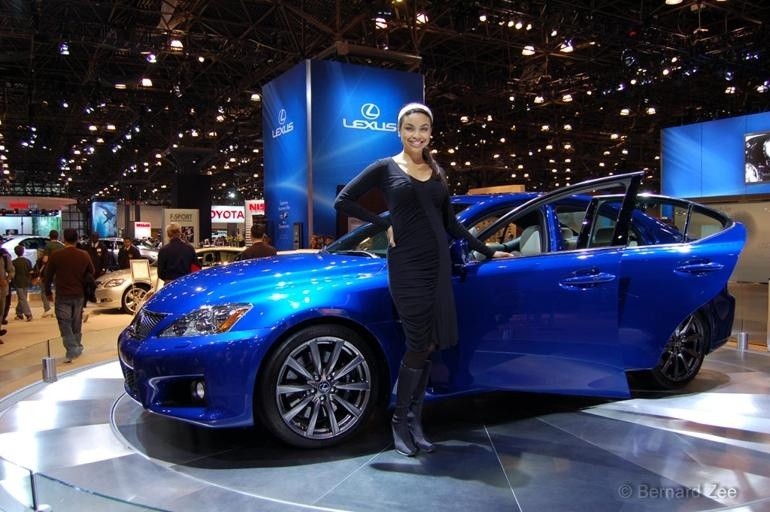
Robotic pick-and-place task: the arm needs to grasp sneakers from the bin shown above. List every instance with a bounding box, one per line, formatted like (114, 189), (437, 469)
(81, 309), (90, 324)
(63, 345), (84, 359)
(1, 319), (9, 325)
(13, 312), (33, 322)
(40, 308), (53, 319)
(0, 329), (7, 337)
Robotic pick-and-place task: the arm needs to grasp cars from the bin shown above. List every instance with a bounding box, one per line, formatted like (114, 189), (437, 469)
(116, 170), (747, 452)
(591, 225), (616, 247)
(0, 235), (250, 315)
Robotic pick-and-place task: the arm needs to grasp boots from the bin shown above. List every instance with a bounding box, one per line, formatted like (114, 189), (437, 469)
(390, 360), (425, 457)
(407, 357), (438, 453)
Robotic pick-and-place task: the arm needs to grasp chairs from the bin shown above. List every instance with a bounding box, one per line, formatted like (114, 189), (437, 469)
(559, 224), (616, 252)
(509, 224), (546, 257)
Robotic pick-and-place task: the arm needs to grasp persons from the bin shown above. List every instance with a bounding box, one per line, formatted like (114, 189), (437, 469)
(44, 228), (96, 363)
(117, 236), (142, 270)
(310, 234), (335, 250)
(156, 222), (202, 285)
(0, 227), (109, 345)
(143, 235), (227, 248)
(240, 224), (277, 260)
(333, 100), (515, 458)
